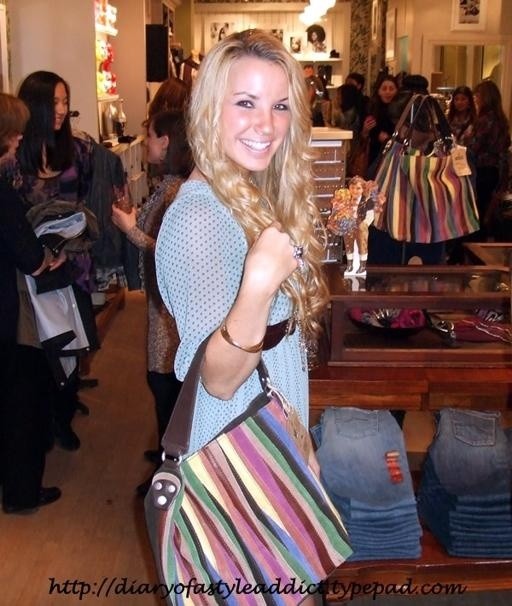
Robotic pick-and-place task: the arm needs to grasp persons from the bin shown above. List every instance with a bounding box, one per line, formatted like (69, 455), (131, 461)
(303, 63), (512, 230)
(0, 92), (67, 516)
(154, 28), (333, 606)
(142, 78), (189, 127)
(343, 175), (375, 273)
(112, 111), (193, 494)
(16, 70), (97, 454)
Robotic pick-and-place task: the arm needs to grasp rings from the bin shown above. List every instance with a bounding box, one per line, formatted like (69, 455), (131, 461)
(294, 244), (304, 258)
(298, 258), (305, 267)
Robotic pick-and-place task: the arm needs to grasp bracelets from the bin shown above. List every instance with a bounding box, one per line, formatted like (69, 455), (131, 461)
(220, 321), (265, 353)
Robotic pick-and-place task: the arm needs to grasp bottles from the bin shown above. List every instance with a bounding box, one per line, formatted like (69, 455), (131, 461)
(114, 98), (126, 136)
(102, 103), (119, 135)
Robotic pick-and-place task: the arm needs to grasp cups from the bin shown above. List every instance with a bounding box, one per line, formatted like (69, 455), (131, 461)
(116, 197), (133, 213)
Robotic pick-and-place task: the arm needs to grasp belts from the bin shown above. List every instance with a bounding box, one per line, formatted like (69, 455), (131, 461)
(262, 317), (301, 352)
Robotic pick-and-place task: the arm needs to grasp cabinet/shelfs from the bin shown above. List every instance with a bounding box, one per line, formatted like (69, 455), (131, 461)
(0, 0), (150, 150)
(303, 123), (357, 267)
(292, 52), (345, 91)
(102, 133), (150, 211)
(302, 258), (511, 439)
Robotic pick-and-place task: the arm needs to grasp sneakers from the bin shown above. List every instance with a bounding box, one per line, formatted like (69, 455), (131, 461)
(144, 449), (161, 462)
(56, 423), (81, 452)
(137, 478), (152, 497)
(4, 487), (63, 514)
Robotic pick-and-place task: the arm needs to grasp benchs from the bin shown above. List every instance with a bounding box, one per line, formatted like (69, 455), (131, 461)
(320, 465), (512, 604)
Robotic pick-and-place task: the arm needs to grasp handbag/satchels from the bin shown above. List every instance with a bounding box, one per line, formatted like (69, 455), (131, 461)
(368, 92), (480, 244)
(143, 327), (354, 606)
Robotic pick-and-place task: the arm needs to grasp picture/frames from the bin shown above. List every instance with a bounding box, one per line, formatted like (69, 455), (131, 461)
(204, 13), (239, 58)
(449, 0), (490, 32)
(282, 30), (308, 56)
